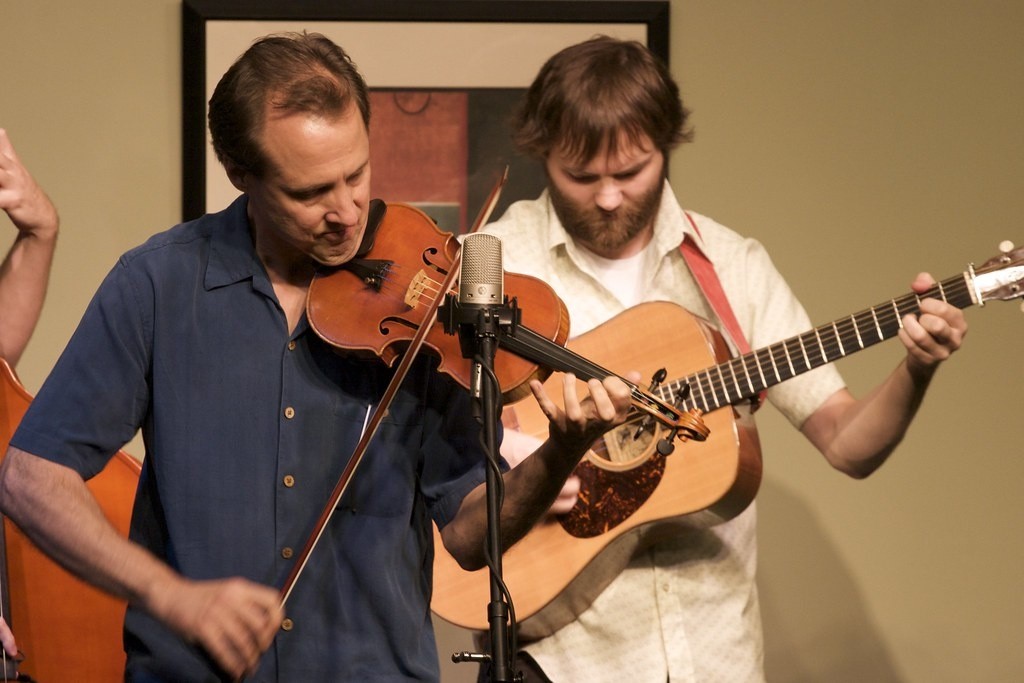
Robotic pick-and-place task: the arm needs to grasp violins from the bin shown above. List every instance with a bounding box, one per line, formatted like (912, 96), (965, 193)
(306, 200), (712, 457)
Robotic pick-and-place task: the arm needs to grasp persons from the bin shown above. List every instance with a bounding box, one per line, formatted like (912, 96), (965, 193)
(0, 127), (61, 656)
(0, 30), (640, 683)
(452, 33), (969, 683)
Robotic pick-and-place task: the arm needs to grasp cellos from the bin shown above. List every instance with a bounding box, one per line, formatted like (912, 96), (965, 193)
(0, 355), (146, 682)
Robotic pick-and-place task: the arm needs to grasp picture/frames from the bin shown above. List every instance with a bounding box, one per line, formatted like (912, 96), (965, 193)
(181, 0), (676, 222)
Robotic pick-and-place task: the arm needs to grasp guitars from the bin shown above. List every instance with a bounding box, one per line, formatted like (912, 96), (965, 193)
(430, 235), (1023, 648)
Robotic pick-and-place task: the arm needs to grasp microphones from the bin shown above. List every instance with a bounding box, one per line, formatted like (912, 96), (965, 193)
(453, 233), (506, 416)
